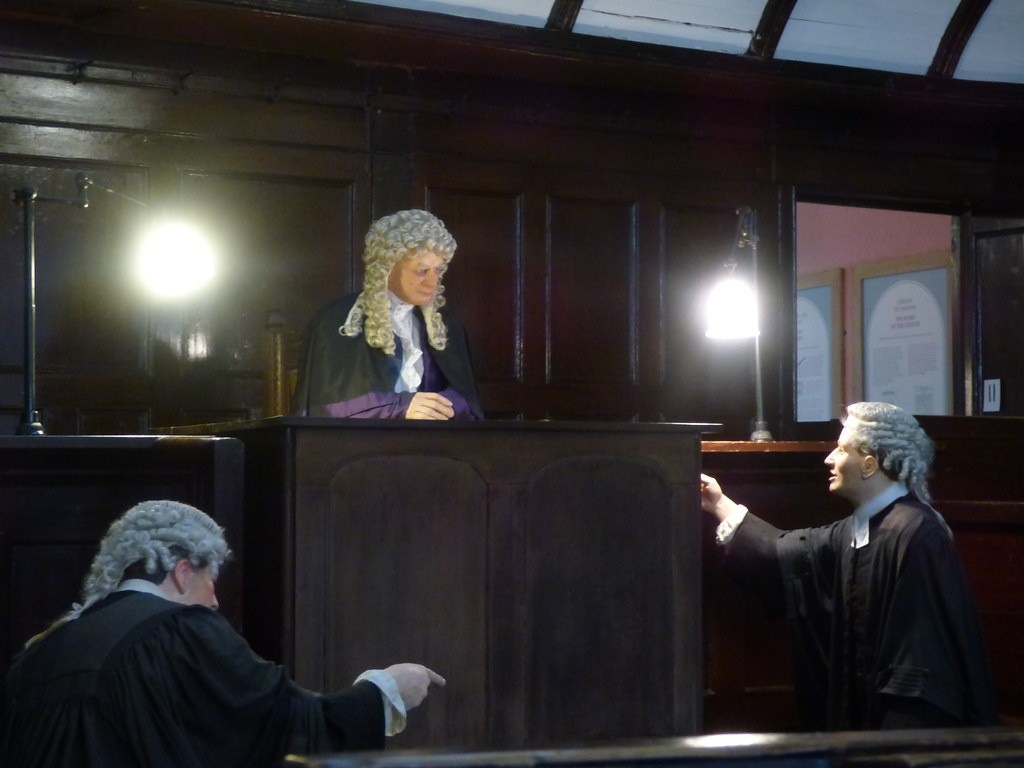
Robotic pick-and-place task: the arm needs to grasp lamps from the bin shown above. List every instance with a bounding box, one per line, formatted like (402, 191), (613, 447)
(11, 172), (228, 436)
(685, 206), (771, 441)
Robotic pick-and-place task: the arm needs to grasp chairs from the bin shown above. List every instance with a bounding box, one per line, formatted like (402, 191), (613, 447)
(262, 308), (305, 418)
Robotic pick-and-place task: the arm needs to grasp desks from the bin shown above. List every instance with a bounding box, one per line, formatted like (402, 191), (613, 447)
(701, 440), (837, 453)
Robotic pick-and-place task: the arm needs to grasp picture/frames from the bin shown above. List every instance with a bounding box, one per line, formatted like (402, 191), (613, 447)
(797, 268), (845, 422)
(851, 250), (954, 416)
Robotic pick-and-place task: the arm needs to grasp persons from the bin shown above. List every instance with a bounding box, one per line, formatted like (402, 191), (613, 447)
(292, 208), (485, 421)
(1, 497), (445, 767)
(700, 401), (999, 732)
